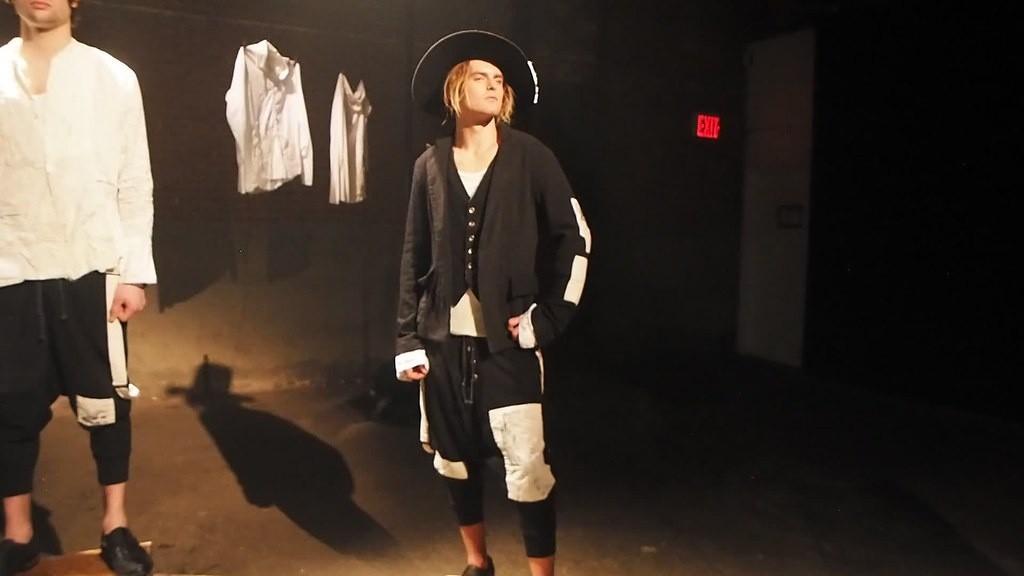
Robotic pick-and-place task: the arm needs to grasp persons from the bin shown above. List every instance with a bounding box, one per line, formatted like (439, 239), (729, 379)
(396, 59), (592, 576)
(0, 0), (154, 576)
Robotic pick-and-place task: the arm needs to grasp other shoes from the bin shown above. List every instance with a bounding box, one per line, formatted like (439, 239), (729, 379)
(462, 555), (495, 576)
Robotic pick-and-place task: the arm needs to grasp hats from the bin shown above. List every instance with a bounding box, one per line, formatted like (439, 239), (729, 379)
(412, 31), (535, 124)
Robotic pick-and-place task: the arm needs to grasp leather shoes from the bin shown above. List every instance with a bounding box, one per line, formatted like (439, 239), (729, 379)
(100, 526), (153, 576)
(0, 534), (42, 576)
